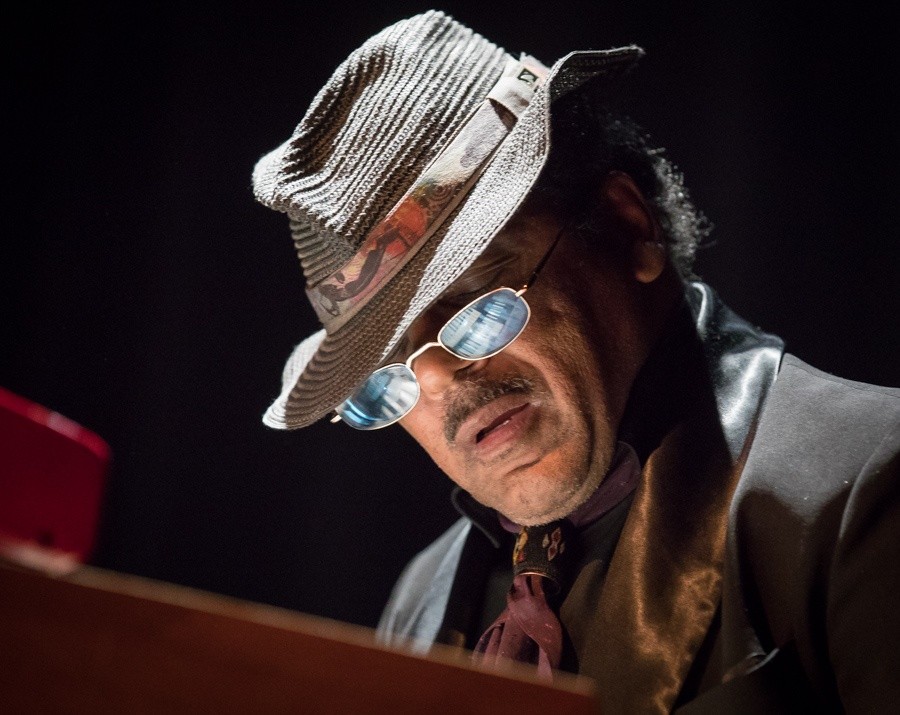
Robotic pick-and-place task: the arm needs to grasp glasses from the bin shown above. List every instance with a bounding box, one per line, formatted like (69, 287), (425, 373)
(330, 209), (577, 432)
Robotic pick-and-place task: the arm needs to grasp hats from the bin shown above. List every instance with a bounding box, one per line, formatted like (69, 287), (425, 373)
(252, 9), (644, 431)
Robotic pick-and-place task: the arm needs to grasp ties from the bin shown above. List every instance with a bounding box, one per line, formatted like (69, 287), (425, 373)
(483, 520), (573, 682)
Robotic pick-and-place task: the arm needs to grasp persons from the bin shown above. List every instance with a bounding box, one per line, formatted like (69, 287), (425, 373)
(252, 9), (900, 715)
